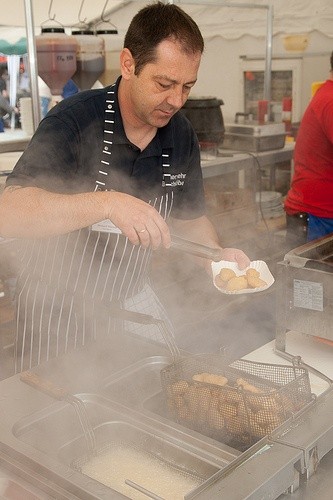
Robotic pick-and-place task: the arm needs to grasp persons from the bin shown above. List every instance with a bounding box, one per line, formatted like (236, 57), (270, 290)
(0, 58), (27, 129)
(0, 0), (251, 379)
(284, 50), (333, 244)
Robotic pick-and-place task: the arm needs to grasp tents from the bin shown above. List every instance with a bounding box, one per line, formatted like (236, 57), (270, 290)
(0, 0), (333, 140)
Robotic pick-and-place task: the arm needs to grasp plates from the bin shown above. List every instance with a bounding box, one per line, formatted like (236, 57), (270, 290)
(257, 190), (284, 220)
(212, 260), (274, 294)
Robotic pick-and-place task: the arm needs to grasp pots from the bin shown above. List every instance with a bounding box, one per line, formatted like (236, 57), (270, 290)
(178, 97), (224, 146)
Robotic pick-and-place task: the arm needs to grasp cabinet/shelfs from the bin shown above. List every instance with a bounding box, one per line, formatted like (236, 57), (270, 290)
(200, 141), (297, 247)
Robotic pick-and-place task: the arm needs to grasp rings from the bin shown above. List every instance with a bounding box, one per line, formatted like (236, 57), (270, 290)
(139, 228), (146, 233)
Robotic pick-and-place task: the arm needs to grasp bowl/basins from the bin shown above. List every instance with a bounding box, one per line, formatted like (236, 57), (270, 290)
(19, 96), (43, 135)
